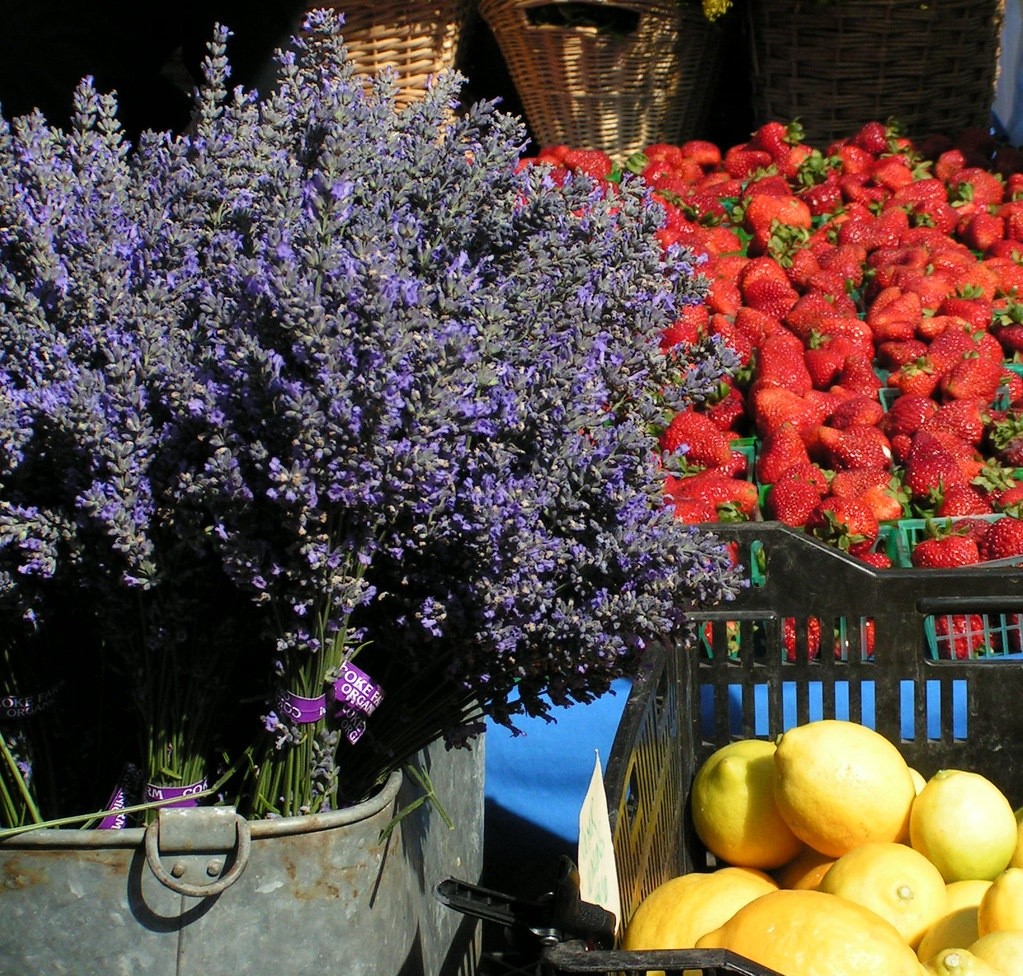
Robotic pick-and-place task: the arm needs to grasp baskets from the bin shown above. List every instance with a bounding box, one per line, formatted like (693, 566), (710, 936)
(663, 361), (1023, 663)
(727, 0), (1006, 163)
(305, 0), (463, 158)
(478, 0), (708, 168)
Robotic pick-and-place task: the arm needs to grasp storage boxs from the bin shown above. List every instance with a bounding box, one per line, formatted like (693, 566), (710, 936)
(441, 518), (1023, 976)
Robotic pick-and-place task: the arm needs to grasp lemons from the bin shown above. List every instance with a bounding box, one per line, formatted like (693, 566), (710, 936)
(620, 719), (1023, 976)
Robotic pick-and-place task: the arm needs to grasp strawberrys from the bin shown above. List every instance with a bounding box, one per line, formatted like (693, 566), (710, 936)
(459, 122), (1023, 662)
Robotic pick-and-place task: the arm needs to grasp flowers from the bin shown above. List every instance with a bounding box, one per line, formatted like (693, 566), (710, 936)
(0, 0), (759, 846)
